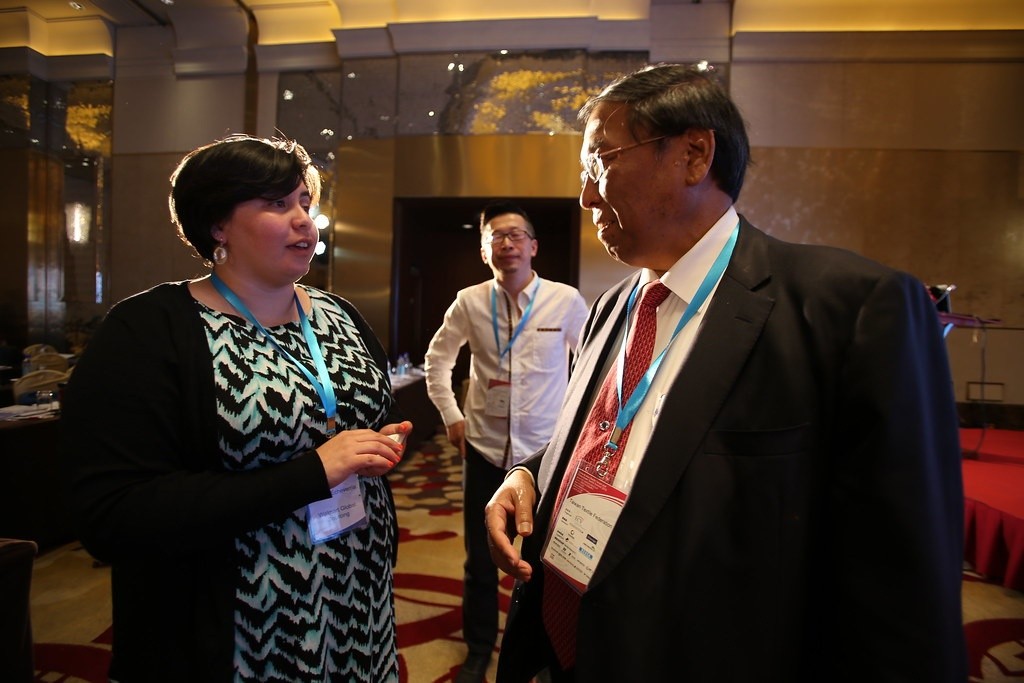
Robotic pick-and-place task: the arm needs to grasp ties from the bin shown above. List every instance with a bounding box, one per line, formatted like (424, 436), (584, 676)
(540, 281), (671, 670)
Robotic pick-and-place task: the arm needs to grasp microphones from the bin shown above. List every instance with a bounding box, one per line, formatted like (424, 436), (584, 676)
(936, 284), (957, 305)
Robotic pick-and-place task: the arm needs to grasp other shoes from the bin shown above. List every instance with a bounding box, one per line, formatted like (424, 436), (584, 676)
(455, 652), (490, 683)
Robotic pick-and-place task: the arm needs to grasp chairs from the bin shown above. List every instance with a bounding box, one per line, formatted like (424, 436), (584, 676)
(23, 344), (55, 356)
(13, 370), (66, 395)
(32, 354), (69, 373)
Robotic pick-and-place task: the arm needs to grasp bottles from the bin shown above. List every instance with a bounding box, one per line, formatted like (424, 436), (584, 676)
(387, 360), (391, 380)
(397, 351), (411, 380)
(21, 354), (32, 377)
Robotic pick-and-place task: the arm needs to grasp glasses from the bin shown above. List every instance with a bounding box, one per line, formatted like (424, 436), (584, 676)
(482, 230), (532, 248)
(579, 133), (673, 188)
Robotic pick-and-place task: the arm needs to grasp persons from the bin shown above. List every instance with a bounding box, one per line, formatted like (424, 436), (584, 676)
(54, 130), (414, 683)
(425, 199), (591, 683)
(485, 58), (972, 683)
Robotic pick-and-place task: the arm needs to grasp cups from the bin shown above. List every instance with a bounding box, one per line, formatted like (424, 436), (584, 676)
(36, 391), (53, 417)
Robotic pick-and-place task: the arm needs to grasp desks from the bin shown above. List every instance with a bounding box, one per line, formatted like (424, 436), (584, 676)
(941, 305), (1024, 340)
(0, 392), (77, 559)
(387, 366), (439, 460)
